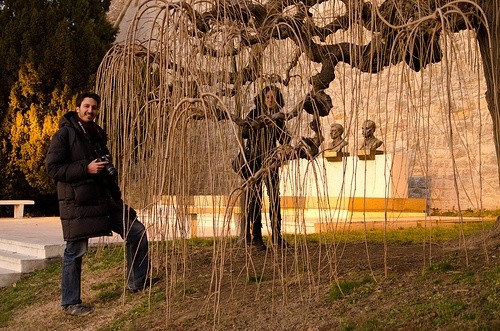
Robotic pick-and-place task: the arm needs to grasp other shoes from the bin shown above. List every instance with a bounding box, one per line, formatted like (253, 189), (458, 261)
(61, 303), (94, 317)
(270, 233), (289, 248)
(251, 235), (266, 250)
(129, 276), (160, 294)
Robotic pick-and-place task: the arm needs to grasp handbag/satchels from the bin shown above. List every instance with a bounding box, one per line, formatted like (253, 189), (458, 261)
(232, 143), (264, 185)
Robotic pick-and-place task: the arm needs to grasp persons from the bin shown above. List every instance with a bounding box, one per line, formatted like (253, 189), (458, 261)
(327, 123), (349, 153)
(360, 119), (383, 152)
(45, 90), (161, 317)
(232, 86), (303, 253)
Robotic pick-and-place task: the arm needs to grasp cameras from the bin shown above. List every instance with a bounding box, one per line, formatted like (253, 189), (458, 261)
(96, 154), (116, 177)
